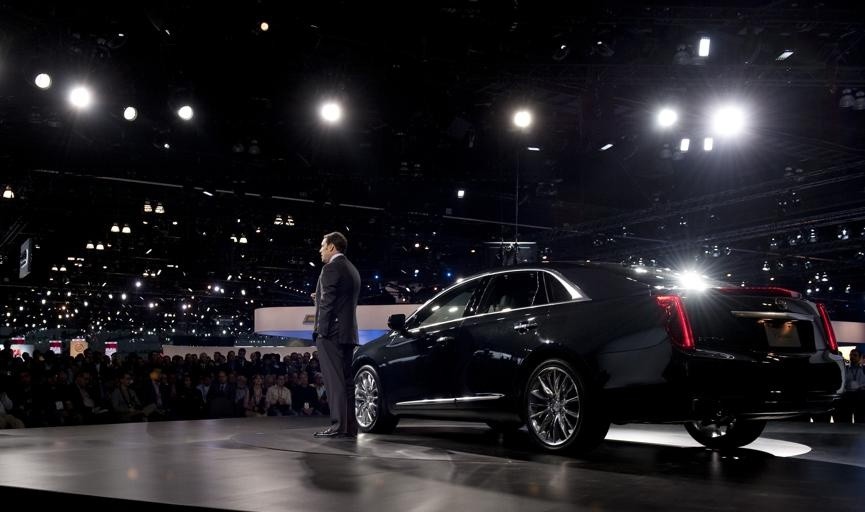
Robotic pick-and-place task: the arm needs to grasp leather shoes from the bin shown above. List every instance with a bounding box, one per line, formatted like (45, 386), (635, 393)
(312, 428), (355, 438)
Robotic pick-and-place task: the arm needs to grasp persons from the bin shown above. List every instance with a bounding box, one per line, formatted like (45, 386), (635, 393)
(0, 340), (328, 428)
(838, 349), (865, 423)
(310, 231), (361, 439)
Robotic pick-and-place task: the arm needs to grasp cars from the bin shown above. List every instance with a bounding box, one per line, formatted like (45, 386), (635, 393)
(353, 259), (848, 455)
